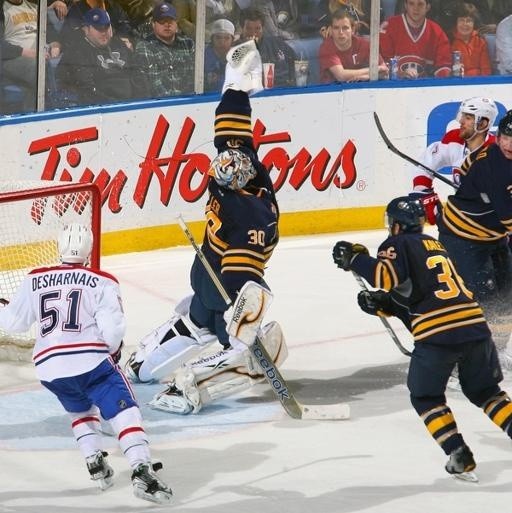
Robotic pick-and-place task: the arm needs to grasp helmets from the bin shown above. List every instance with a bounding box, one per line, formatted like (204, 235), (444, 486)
(207, 149), (258, 195)
(496, 109), (512, 138)
(458, 94), (499, 135)
(57, 223), (95, 264)
(385, 196), (425, 229)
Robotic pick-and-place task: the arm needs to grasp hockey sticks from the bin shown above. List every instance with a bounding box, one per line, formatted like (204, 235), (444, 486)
(353, 272), (412, 355)
(373, 112), (459, 188)
(175, 210), (349, 421)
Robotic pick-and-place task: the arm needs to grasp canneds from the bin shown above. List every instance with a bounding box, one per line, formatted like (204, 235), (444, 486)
(390, 58), (399, 81)
(452, 51), (465, 76)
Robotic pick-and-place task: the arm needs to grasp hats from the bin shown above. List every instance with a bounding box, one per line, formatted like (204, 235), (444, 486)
(152, 4), (177, 22)
(84, 8), (112, 26)
(209, 18), (235, 36)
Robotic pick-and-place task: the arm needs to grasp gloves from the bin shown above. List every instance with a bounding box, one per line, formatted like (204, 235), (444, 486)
(416, 192), (444, 226)
(332, 240), (370, 271)
(355, 288), (389, 320)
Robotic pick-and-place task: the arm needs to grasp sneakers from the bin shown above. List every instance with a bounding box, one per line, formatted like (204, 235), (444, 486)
(443, 447), (482, 485)
(84, 453), (115, 489)
(130, 462), (176, 507)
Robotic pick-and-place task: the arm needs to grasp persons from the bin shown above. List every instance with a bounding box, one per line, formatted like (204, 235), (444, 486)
(333, 195), (512, 476)
(127, 39), (284, 418)
(434, 109), (511, 299)
(1, 220), (174, 504)
(1, 1), (512, 115)
(413, 94), (498, 224)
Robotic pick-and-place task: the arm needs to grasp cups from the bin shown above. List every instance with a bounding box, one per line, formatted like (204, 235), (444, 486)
(294, 60), (309, 87)
(262, 62), (275, 89)
